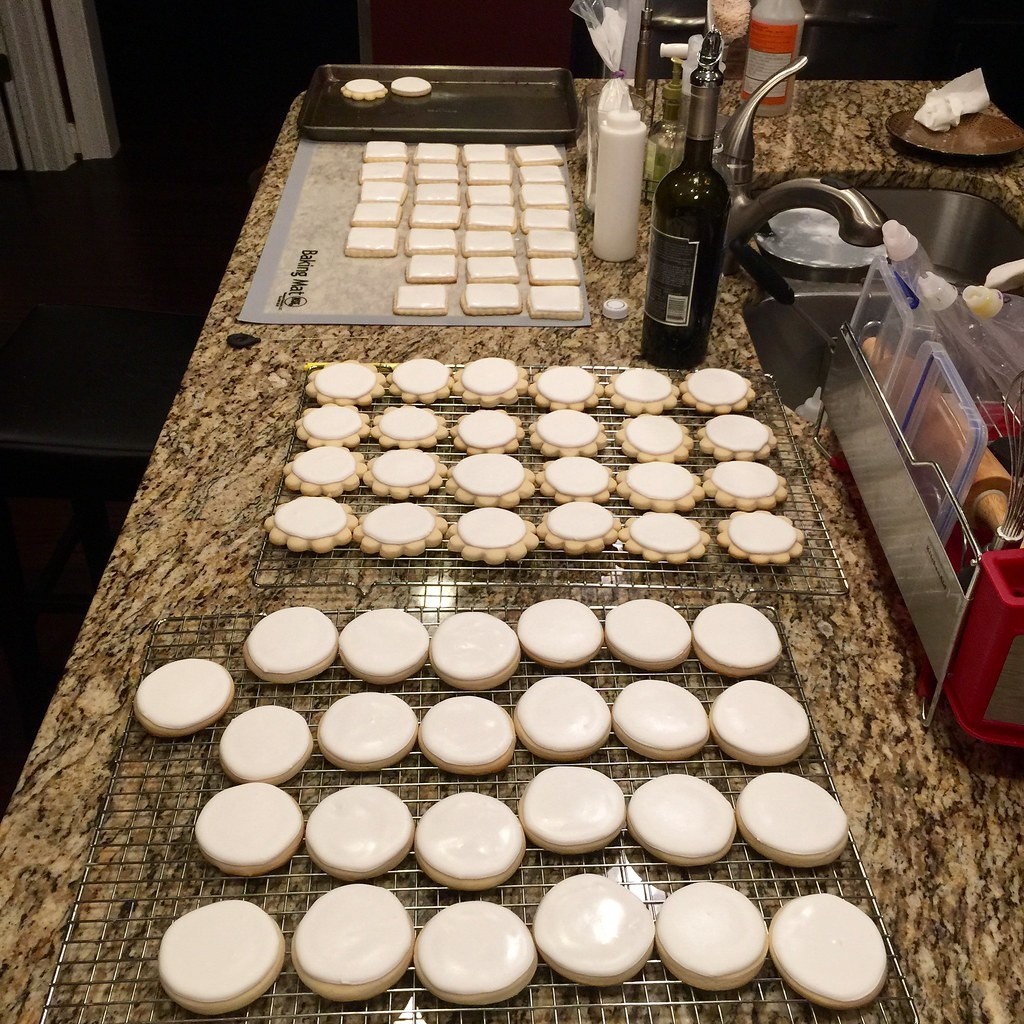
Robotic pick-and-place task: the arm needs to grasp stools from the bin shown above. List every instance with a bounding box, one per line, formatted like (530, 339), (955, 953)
(0, 302), (208, 752)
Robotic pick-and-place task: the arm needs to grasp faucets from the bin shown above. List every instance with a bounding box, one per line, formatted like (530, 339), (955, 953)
(717, 53), (888, 321)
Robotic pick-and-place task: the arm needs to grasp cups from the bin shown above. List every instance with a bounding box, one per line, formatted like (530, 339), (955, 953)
(584, 94), (647, 213)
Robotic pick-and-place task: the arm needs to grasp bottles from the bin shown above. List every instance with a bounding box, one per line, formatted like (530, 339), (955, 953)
(740, 0), (805, 117)
(592, 93), (647, 262)
(644, 58), (687, 203)
(795, 388), (829, 427)
(640, 31), (732, 370)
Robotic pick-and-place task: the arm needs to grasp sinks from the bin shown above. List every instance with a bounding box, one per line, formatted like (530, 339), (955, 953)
(726, 293), (890, 422)
(745, 170), (1024, 291)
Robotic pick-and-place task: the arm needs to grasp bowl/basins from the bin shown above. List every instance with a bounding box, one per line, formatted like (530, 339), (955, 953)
(754, 221), (888, 284)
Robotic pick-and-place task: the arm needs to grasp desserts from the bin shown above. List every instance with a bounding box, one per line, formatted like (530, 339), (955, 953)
(133, 76), (887, 1008)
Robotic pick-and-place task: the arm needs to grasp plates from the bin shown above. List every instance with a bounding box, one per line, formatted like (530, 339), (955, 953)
(886, 110), (1024, 165)
